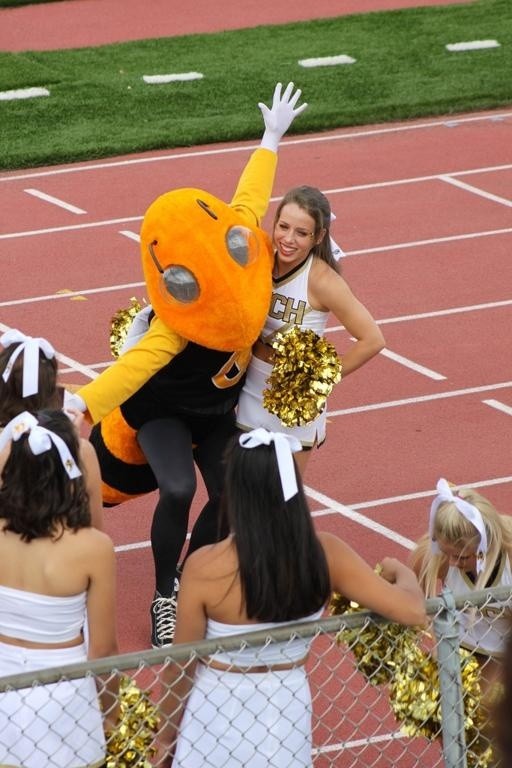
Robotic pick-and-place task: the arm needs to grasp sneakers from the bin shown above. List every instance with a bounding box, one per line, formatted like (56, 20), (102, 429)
(149, 590), (178, 649)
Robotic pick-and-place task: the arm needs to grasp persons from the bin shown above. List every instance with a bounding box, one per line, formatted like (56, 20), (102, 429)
(0, 406), (126, 768)
(0, 319), (105, 536)
(59, 79), (309, 655)
(145, 427), (427, 768)
(234, 182), (387, 490)
(408, 475), (511, 763)
